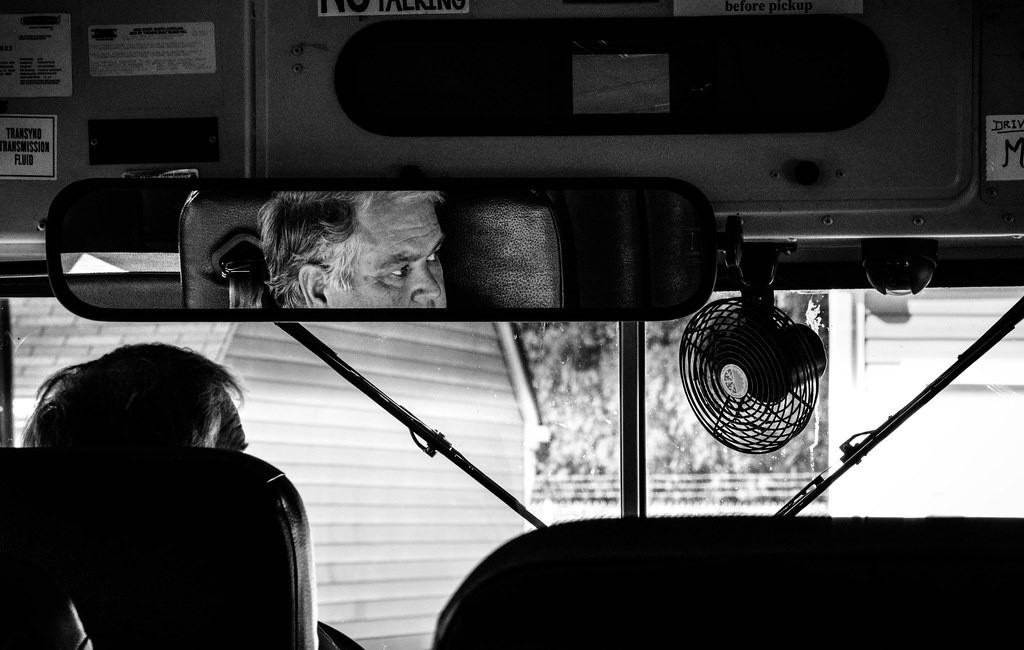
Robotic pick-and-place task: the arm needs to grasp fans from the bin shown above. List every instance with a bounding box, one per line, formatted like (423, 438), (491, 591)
(677, 238), (826, 454)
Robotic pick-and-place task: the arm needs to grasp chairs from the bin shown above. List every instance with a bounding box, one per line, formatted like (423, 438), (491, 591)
(0, 443), (315, 650)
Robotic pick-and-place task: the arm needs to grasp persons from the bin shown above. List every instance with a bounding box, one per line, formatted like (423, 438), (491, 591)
(258, 191), (447, 310)
(23, 342), (340, 650)
